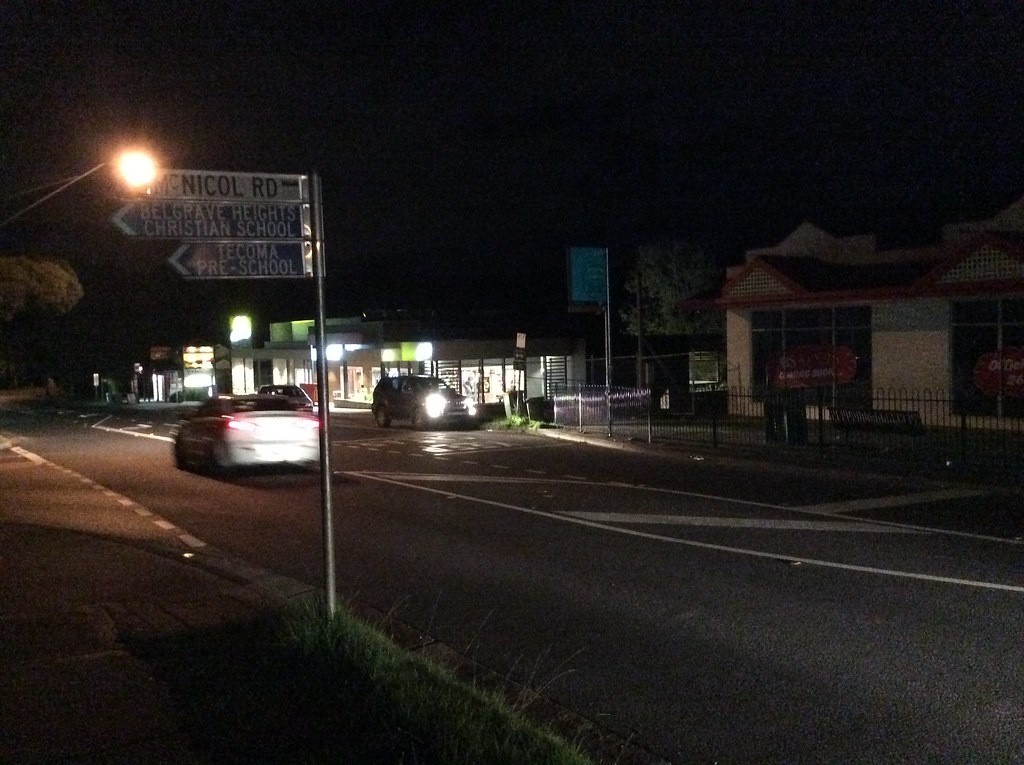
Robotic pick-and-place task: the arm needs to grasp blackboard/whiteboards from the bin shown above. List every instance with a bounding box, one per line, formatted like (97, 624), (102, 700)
(122, 392), (139, 403)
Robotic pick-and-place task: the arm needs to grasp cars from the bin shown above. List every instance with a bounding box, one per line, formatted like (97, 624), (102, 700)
(173, 384), (319, 480)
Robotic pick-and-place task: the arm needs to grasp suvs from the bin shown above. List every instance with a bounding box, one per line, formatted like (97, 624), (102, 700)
(371, 376), (480, 430)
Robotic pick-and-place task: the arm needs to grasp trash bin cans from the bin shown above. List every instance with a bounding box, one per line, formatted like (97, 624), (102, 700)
(526, 397), (545, 422)
(503, 390), (524, 418)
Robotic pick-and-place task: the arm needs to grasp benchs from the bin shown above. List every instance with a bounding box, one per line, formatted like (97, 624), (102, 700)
(827, 406), (938, 452)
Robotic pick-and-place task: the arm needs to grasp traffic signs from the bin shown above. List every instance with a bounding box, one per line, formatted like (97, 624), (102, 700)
(101, 153), (325, 283)
